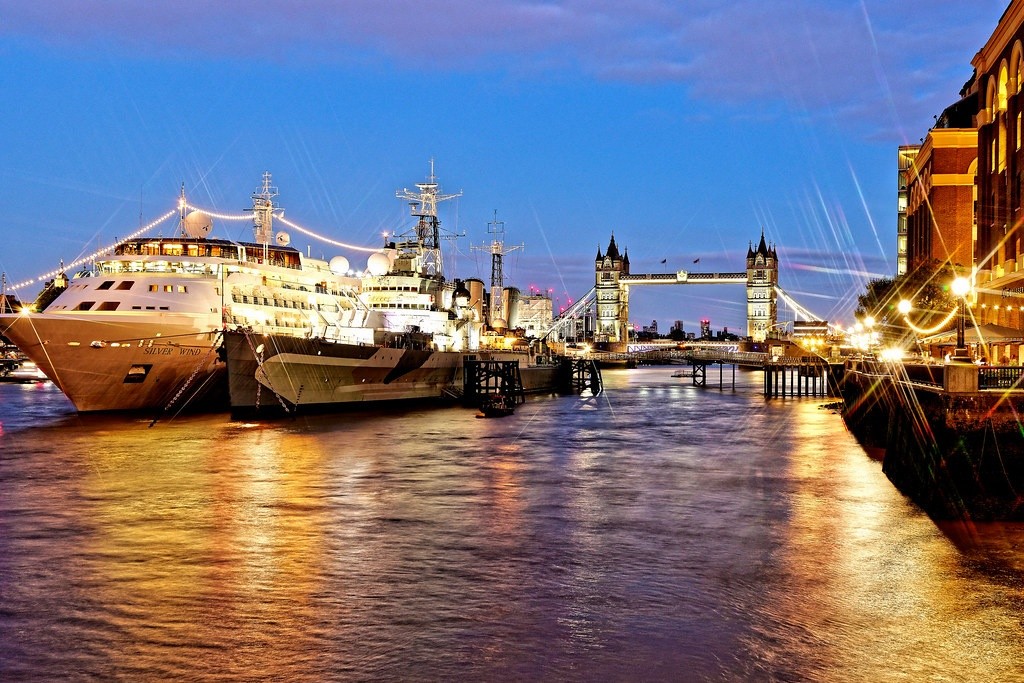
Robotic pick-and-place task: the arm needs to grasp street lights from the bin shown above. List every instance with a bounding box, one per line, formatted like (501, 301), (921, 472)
(941, 276), (980, 393)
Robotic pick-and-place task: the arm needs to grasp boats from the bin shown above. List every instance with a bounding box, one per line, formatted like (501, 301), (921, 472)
(0, 154), (587, 422)
